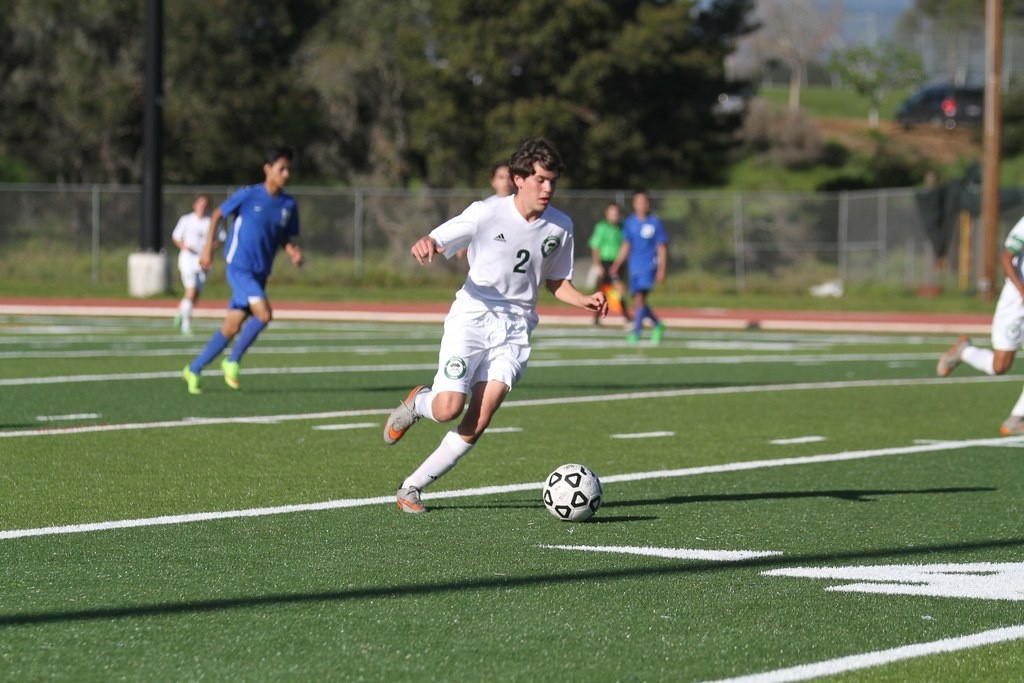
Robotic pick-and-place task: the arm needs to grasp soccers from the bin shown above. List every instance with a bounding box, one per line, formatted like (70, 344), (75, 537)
(542, 462), (602, 521)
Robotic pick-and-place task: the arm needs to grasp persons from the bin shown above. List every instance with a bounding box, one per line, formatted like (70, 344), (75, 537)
(382, 140), (609, 513)
(456, 158), (515, 258)
(171, 194), (226, 334)
(181, 143), (305, 395)
(587, 202), (632, 326)
(936, 214), (1024, 434)
(609, 189), (669, 344)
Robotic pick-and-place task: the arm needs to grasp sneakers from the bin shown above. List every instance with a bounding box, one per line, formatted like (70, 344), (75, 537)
(999, 419), (1024, 435)
(220, 356), (240, 389)
(396, 483), (428, 512)
(626, 335), (640, 341)
(174, 315), (182, 327)
(936, 336), (972, 376)
(183, 364), (203, 394)
(182, 326), (194, 337)
(383, 385), (432, 445)
(650, 322), (665, 342)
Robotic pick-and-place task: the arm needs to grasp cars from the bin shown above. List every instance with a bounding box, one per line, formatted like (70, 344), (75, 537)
(900, 80), (984, 125)
